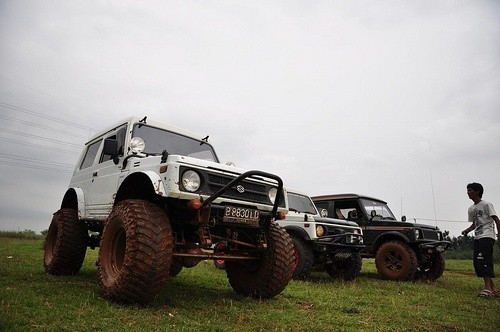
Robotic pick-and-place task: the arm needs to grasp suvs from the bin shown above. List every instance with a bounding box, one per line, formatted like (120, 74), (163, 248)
(214, 188), (366, 279)
(43, 115), (296, 303)
(310, 194), (451, 280)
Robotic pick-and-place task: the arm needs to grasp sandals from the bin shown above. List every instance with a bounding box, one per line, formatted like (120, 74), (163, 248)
(478, 288), (496, 297)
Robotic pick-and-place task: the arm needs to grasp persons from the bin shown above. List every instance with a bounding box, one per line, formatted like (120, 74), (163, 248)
(461, 182), (500, 297)
